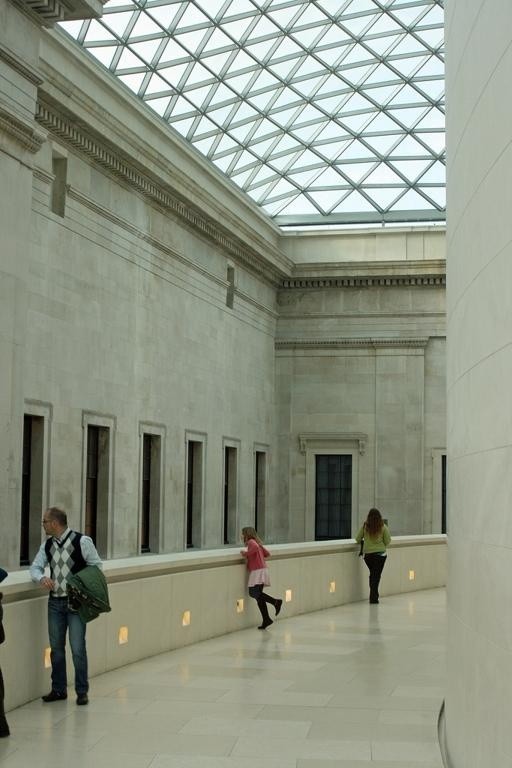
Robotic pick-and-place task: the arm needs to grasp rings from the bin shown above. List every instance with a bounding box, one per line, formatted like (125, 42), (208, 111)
(44, 583), (47, 585)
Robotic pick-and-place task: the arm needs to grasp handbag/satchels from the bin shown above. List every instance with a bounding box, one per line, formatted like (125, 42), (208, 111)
(358, 522), (365, 556)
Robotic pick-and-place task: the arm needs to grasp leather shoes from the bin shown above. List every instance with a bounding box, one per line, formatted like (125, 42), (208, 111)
(275, 599), (282, 616)
(41, 691), (67, 702)
(257, 617), (273, 629)
(76, 693), (88, 705)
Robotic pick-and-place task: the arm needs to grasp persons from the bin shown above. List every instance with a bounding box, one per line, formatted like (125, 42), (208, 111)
(28, 507), (103, 705)
(355, 508), (391, 604)
(240, 526), (282, 629)
(0, 568), (10, 737)
